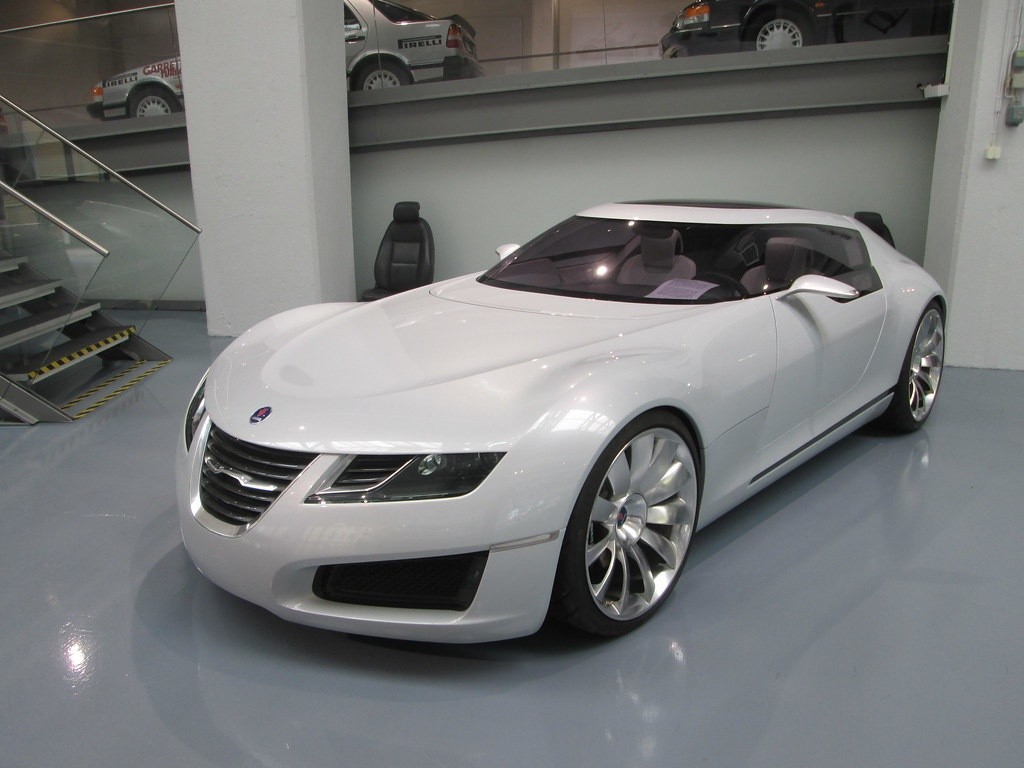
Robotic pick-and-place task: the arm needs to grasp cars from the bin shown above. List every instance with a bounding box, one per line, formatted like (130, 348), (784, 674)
(87, 0), (480, 121)
(661, 0), (954, 61)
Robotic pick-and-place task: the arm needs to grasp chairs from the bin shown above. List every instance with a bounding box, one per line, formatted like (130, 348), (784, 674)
(357, 201), (436, 303)
(735, 237), (827, 297)
(617, 230), (696, 287)
(853, 211), (895, 249)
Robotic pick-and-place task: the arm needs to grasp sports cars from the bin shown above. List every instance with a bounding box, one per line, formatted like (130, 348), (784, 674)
(171, 201), (958, 643)
